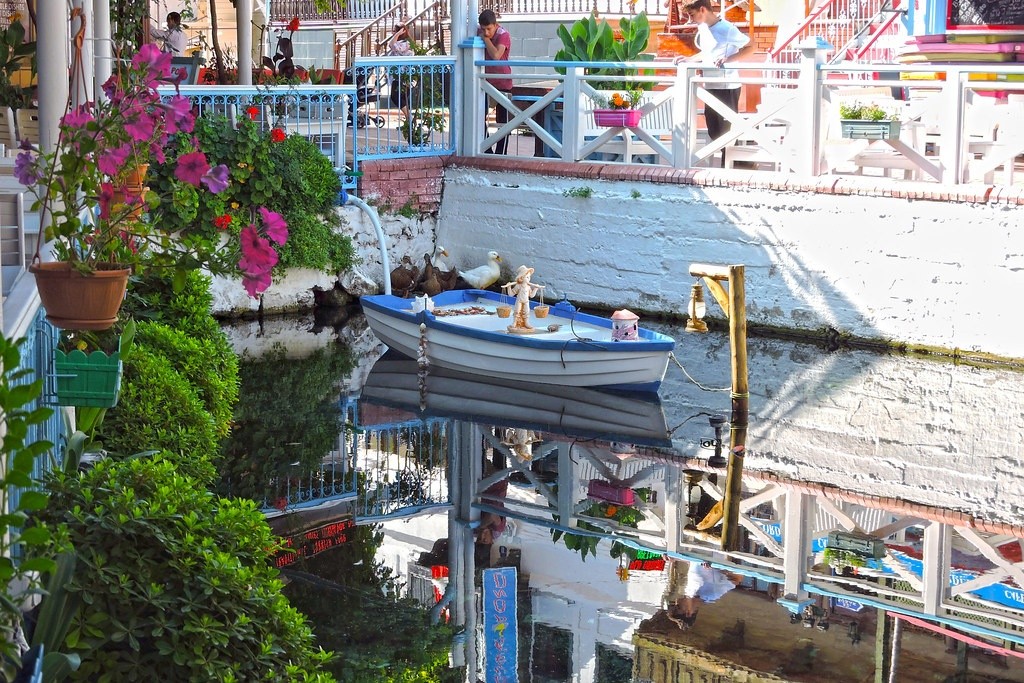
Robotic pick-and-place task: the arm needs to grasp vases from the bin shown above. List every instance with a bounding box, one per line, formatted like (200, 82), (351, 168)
(586, 480), (636, 506)
(27, 260), (131, 332)
(593, 109), (643, 129)
(54, 347), (125, 410)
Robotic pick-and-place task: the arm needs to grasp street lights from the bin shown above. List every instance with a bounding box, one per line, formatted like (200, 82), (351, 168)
(682, 399), (749, 552)
(685, 262), (753, 398)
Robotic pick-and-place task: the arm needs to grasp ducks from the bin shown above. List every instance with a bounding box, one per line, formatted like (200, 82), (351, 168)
(457, 251), (503, 291)
(390, 245), (458, 299)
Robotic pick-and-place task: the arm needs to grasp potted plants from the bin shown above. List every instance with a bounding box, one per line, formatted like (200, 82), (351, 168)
(838, 101), (903, 141)
(0, 20), (43, 150)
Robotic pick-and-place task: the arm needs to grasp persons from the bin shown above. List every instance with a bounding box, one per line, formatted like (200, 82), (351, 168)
(475, 425), (509, 558)
(661, 552), (744, 631)
(477, 9), (513, 155)
(389, 22), (415, 131)
(506, 428), (540, 461)
(506, 265), (539, 329)
(672, 0), (758, 170)
(150, 12), (188, 57)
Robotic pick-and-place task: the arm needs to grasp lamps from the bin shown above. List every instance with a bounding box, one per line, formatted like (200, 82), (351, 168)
(684, 280), (709, 333)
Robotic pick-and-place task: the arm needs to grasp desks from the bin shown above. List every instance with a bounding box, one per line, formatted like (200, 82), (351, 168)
(489, 87), (564, 156)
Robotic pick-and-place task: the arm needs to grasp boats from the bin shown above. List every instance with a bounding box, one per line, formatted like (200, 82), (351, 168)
(359, 360), (673, 448)
(360, 288), (677, 393)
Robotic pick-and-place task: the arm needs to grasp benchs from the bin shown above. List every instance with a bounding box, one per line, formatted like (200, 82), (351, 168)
(580, 85), (1024, 184)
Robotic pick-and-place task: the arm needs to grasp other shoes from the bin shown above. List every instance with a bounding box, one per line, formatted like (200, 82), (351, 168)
(396, 126), (404, 131)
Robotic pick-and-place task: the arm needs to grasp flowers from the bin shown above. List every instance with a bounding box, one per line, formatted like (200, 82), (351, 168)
(15, 17), (308, 352)
(563, 499), (648, 583)
(589, 86), (645, 111)
